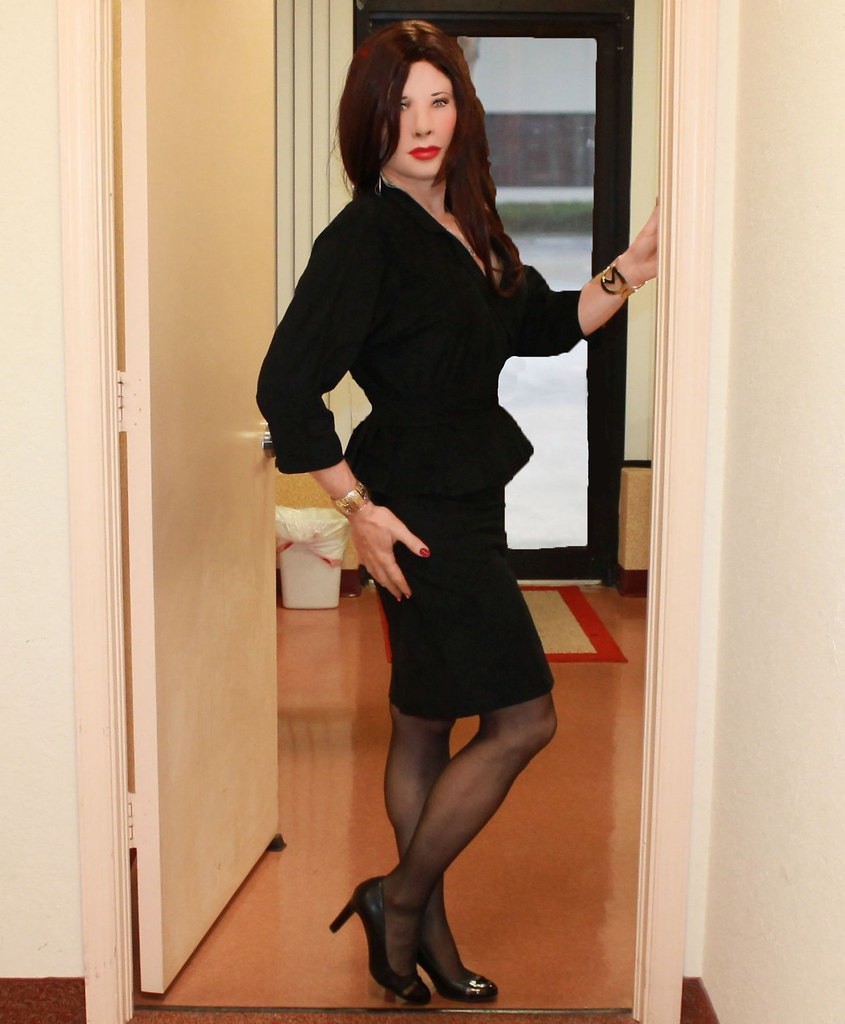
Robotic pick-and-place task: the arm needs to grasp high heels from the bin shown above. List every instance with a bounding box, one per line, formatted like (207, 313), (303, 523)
(329, 875), (499, 1005)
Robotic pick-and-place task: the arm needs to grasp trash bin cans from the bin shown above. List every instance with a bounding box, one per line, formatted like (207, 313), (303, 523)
(275, 507), (350, 609)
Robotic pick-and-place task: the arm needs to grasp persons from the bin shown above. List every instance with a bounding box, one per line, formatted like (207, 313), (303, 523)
(253, 19), (661, 1010)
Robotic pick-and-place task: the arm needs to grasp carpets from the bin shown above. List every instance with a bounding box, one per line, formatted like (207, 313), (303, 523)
(373, 585), (629, 664)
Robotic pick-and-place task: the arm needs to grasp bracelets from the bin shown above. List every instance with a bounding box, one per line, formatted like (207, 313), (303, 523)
(595, 255), (646, 301)
(329, 478), (371, 517)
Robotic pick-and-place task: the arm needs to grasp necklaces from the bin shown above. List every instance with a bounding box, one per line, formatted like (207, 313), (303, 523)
(425, 205), (478, 257)
